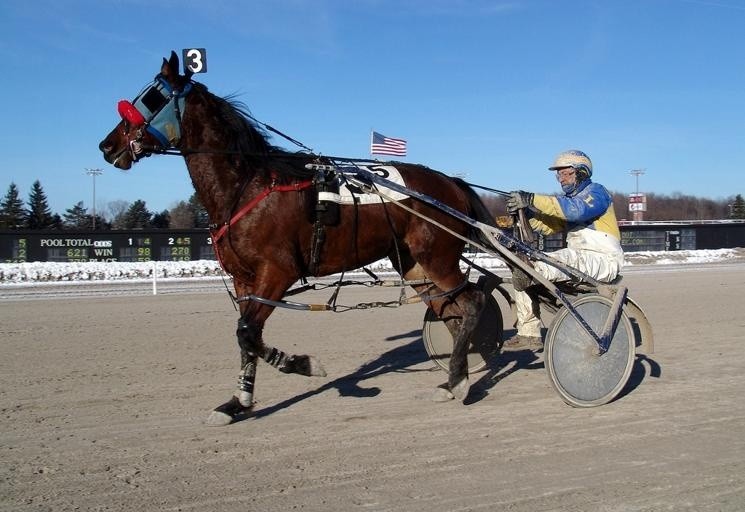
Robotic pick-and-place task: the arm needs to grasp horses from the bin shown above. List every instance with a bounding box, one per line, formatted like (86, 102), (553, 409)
(97, 49), (503, 429)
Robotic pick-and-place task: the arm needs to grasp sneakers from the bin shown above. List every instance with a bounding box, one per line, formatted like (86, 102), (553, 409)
(498, 335), (544, 352)
(510, 251), (534, 292)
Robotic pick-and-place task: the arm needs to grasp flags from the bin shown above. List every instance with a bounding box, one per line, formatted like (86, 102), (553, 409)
(371, 130), (406, 156)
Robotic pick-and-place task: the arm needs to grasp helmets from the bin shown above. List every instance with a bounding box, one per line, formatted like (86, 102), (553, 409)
(549, 149), (594, 184)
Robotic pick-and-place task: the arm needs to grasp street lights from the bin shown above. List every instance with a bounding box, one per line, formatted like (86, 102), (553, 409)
(629, 168), (645, 224)
(85, 168), (104, 230)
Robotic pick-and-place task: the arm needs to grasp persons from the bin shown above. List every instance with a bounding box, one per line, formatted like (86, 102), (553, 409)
(502, 150), (624, 351)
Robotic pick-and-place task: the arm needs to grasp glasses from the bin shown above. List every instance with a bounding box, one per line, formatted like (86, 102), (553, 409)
(553, 171), (575, 181)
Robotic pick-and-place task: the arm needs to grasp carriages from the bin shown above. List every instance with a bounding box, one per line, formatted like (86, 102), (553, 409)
(99, 51), (655, 427)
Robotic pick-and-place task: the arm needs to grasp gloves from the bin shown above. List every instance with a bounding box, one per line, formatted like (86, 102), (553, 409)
(505, 190), (535, 218)
